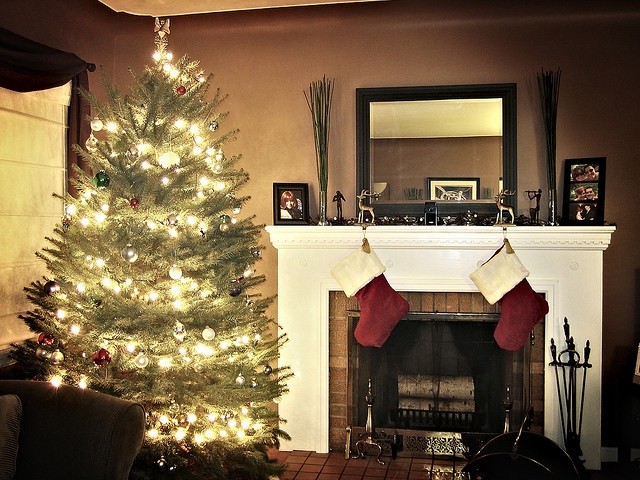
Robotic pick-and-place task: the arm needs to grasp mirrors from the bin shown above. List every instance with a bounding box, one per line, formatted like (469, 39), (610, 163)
(355, 80), (518, 219)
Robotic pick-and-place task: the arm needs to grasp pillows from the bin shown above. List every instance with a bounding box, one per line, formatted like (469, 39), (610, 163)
(0, 393), (28, 480)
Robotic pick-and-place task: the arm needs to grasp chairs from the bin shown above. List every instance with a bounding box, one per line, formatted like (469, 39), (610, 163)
(28, 385), (146, 479)
(0, 380), (51, 480)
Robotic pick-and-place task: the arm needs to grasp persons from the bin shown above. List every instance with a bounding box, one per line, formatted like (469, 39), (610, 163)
(583, 164), (599, 180)
(278, 190), (303, 220)
(576, 204), (597, 220)
(572, 168), (586, 180)
(573, 186), (585, 200)
(586, 186), (597, 199)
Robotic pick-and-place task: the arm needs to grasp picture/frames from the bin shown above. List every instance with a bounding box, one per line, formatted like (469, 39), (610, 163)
(273, 182), (309, 225)
(427, 177), (481, 201)
(562, 156), (605, 226)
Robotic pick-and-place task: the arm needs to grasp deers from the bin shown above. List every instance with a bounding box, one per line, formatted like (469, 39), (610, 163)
(493, 190), (515, 225)
(357, 189), (376, 223)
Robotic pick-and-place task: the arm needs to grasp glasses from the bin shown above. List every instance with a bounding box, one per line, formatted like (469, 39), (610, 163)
(575, 172), (584, 177)
(584, 169), (593, 174)
(586, 191), (592, 194)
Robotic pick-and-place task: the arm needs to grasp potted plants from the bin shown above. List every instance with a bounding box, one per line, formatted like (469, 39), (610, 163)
(536, 64), (563, 227)
(303, 73), (338, 228)
